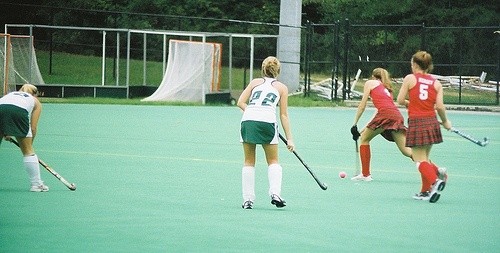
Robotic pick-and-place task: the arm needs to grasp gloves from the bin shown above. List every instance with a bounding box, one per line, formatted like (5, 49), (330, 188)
(351, 125), (361, 140)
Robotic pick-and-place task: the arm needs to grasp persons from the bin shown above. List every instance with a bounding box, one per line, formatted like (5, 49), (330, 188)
(0, 83), (49, 193)
(398, 51), (451, 202)
(237, 56), (293, 208)
(350, 68), (448, 186)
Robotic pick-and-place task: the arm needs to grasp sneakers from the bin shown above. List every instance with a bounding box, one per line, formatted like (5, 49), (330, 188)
(438, 168), (448, 181)
(31, 181), (48, 192)
(413, 192), (431, 201)
(429, 179), (446, 203)
(242, 201), (254, 209)
(351, 174), (372, 182)
(271, 194), (287, 208)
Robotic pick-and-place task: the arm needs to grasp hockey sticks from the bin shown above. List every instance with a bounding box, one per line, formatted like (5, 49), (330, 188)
(11, 137), (76, 191)
(439, 120), (489, 147)
(355, 140), (361, 176)
(279, 133), (328, 190)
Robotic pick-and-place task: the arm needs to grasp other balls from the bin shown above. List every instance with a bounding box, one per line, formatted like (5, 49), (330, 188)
(339, 172), (346, 178)
